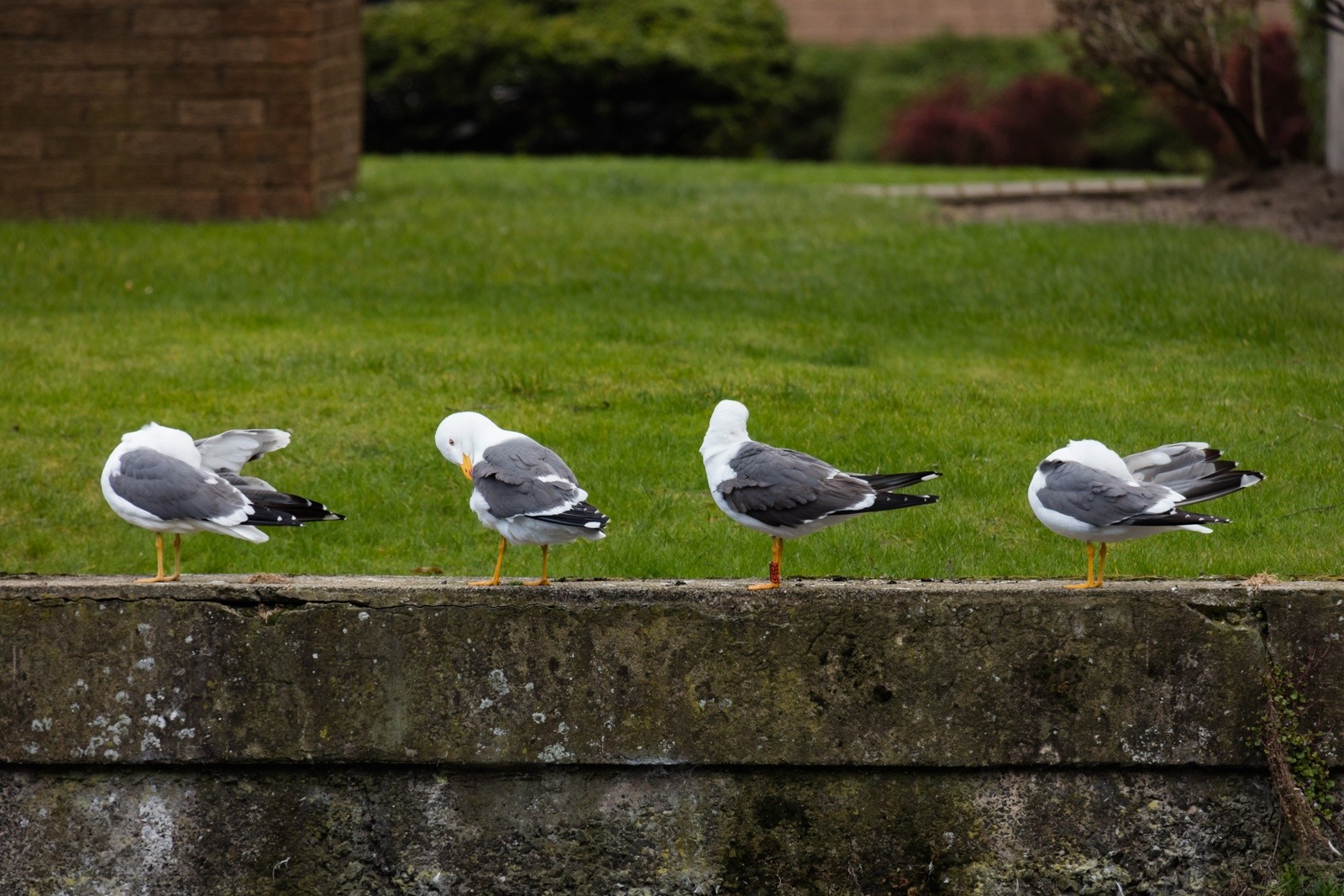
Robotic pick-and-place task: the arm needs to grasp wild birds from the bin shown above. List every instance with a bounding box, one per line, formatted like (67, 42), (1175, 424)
(1027, 437), (1267, 589)
(699, 399), (944, 592)
(433, 410), (610, 585)
(100, 421), (348, 582)
